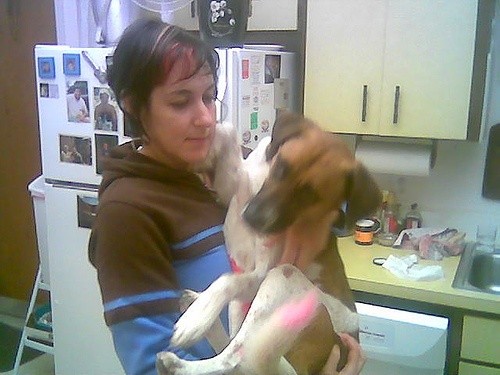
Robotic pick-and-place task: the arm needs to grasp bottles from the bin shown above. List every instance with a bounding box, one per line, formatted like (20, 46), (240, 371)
(405, 201), (423, 229)
(386, 190), (399, 232)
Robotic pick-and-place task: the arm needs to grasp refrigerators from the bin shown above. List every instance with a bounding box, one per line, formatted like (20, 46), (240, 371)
(35, 42), (296, 375)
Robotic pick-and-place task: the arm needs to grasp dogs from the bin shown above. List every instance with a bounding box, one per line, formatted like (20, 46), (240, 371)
(154, 109), (383, 375)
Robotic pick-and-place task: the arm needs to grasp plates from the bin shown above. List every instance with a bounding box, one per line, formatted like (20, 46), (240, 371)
(241, 44), (286, 51)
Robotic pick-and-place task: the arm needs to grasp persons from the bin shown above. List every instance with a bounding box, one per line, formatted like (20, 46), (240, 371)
(86, 15), (367, 374)
(70, 147), (82, 162)
(61, 145), (71, 162)
(101, 140), (111, 155)
(94, 90), (117, 132)
(42, 62), (50, 74)
(66, 61), (74, 71)
(66, 87), (88, 123)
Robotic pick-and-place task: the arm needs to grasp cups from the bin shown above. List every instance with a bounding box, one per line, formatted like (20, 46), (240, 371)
(477, 223), (496, 253)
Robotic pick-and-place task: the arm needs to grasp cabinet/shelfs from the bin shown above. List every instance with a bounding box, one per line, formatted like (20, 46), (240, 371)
(353, 302), (449, 375)
(297, 0), (495, 142)
(458, 314), (500, 375)
